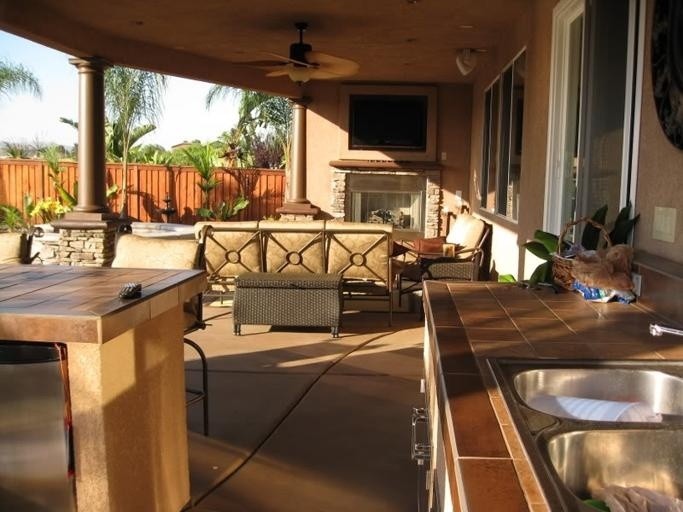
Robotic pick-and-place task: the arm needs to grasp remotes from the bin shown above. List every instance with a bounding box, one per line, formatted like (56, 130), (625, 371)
(119, 282), (141, 298)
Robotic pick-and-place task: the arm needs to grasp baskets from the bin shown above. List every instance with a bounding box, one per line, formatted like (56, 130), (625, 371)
(551, 216), (614, 293)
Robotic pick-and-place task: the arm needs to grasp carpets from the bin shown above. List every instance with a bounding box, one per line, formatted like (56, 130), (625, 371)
(210, 288), (410, 313)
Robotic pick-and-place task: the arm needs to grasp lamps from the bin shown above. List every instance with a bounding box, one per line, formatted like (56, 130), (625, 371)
(452, 47), (489, 77)
(286, 69), (306, 87)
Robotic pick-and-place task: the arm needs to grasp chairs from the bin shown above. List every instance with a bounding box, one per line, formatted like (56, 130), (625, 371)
(0, 229), (41, 265)
(106, 232), (213, 440)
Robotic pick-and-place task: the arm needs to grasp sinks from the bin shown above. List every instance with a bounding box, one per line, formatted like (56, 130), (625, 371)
(526, 426), (683, 512)
(485, 350), (683, 427)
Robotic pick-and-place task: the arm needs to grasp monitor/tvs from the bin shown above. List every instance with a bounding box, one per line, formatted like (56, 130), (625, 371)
(350, 94), (428, 150)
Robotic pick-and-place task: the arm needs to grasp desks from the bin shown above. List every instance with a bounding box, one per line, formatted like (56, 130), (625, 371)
(0, 262), (211, 512)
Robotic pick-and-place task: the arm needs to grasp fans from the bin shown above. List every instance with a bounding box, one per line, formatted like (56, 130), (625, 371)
(264, 22), (358, 81)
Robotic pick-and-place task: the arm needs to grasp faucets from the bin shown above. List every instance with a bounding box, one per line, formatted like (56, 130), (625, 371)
(649, 321), (683, 341)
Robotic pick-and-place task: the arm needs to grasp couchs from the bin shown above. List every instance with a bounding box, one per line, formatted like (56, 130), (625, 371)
(393, 211), (491, 310)
(191, 220), (405, 329)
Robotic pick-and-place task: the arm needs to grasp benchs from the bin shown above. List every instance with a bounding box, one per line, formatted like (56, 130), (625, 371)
(231, 273), (341, 340)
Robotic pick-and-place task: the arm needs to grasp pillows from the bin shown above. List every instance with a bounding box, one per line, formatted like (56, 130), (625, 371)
(387, 235), (451, 261)
